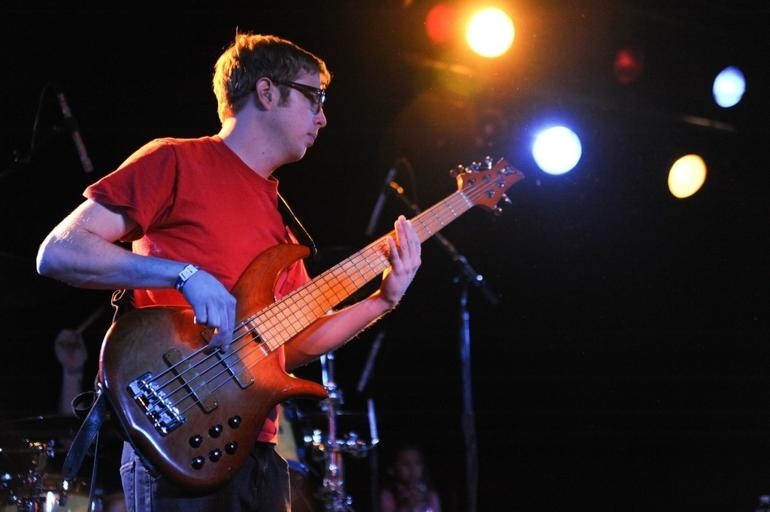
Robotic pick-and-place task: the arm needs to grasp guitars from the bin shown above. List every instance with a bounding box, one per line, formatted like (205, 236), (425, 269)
(98, 157), (526, 498)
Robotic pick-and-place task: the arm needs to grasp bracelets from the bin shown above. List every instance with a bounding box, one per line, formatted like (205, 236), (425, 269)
(175, 264), (199, 291)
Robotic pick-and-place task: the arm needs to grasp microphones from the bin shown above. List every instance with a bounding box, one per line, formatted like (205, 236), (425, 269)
(56, 92), (95, 173)
(365, 166), (396, 237)
(357, 333), (385, 391)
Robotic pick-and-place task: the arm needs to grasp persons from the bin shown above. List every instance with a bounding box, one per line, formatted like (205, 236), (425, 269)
(365, 440), (441, 511)
(36, 32), (424, 512)
(53, 327), (90, 421)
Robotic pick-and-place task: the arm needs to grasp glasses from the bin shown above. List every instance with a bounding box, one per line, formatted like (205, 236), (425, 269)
(276, 76), (326, 104)
